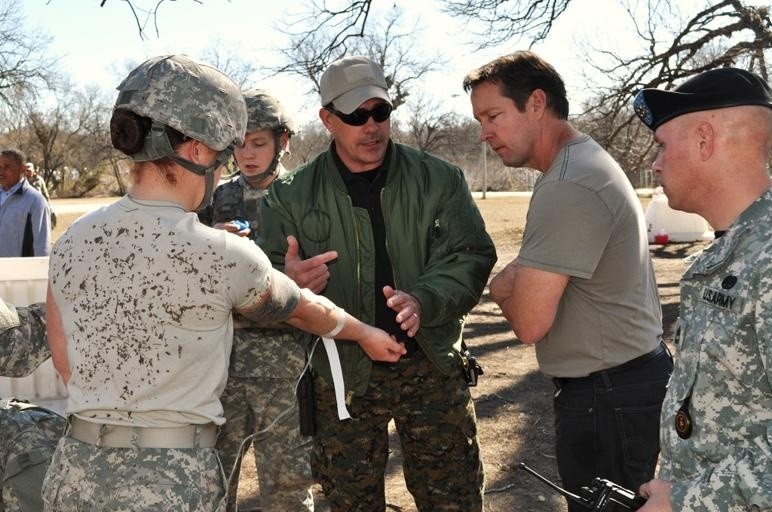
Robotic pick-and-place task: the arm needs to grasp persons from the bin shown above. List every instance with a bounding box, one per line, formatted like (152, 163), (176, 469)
(25, 162), (49, 201)
(463, 50), (673, 512)
(636, 68), (772, 512)
(40, 53), (405, 512)
(198, 87), (315, 512)
(256, 56), (498, 512)
(0, 296), (67, 512)
(0, 149), (50, 259)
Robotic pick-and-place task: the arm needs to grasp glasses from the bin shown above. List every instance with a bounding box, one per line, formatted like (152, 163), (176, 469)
(327, 102), (392, 126)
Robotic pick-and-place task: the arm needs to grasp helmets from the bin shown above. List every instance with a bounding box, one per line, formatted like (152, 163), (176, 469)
(111, 55), (247, 150)
(241, 87), (296, 136)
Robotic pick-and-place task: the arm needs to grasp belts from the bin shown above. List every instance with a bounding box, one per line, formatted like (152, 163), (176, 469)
(552, 340), (665, 390)
(65, 416), (215, 448)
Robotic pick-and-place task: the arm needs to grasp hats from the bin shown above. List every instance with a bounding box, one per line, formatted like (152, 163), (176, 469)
(633, 66), (772, 128)
(320, 54), (393, 115)
